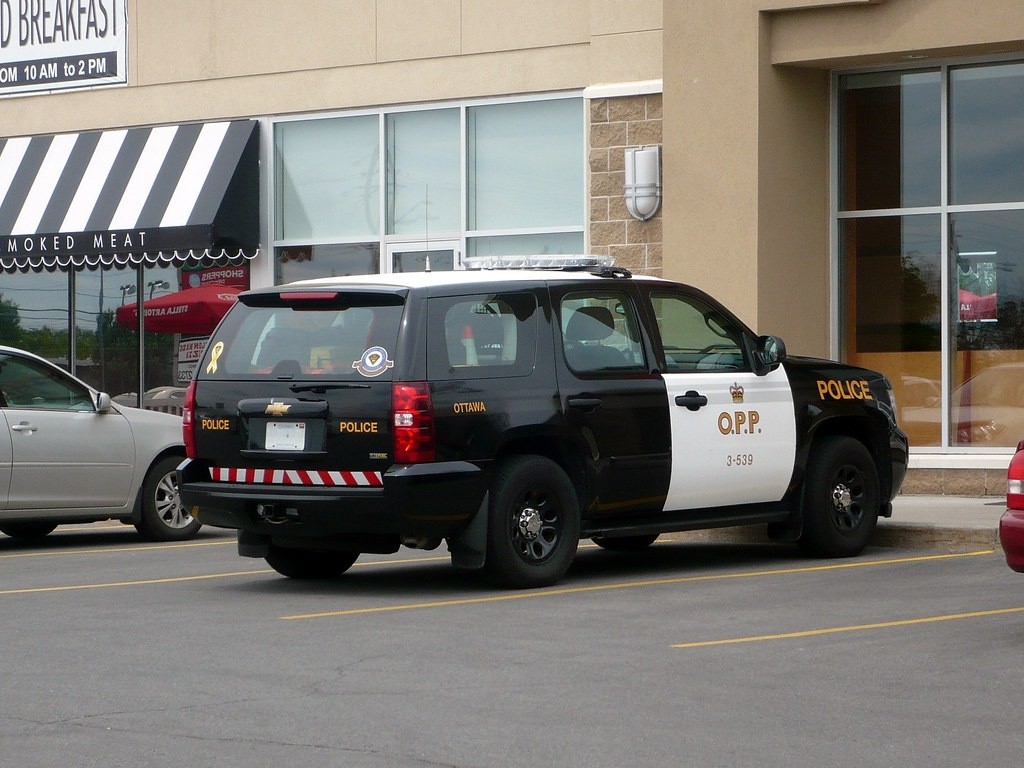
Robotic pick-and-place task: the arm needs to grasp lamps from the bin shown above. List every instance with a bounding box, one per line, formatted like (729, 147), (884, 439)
(623, 144), (661, 221)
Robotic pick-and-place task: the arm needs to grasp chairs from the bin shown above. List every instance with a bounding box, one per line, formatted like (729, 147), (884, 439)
(565, 306), (627, 370)
(447, 312), (504, 362)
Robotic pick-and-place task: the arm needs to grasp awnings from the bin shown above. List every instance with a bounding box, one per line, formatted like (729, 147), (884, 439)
(1, 120), (260, 267)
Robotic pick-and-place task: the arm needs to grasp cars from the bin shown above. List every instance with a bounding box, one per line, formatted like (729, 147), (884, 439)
(111, 384), (187, 400)
(997, 439), (1024, 572)
(0, 343), (203, 542)
(902, 360), (1024, 450)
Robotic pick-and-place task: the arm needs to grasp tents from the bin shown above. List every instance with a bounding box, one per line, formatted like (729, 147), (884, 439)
(117, 283), (244, 333)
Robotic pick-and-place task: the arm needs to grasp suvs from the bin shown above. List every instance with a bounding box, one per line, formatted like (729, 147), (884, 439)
(168, 251), (909, 593)
